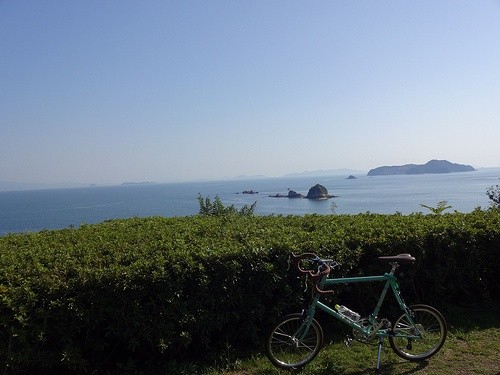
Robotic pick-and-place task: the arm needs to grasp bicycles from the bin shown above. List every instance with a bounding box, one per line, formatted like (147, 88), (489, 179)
(265, 248), (448, 370)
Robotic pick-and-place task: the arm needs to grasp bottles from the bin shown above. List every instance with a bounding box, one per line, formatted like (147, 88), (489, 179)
(335, 304), (361, 323)
